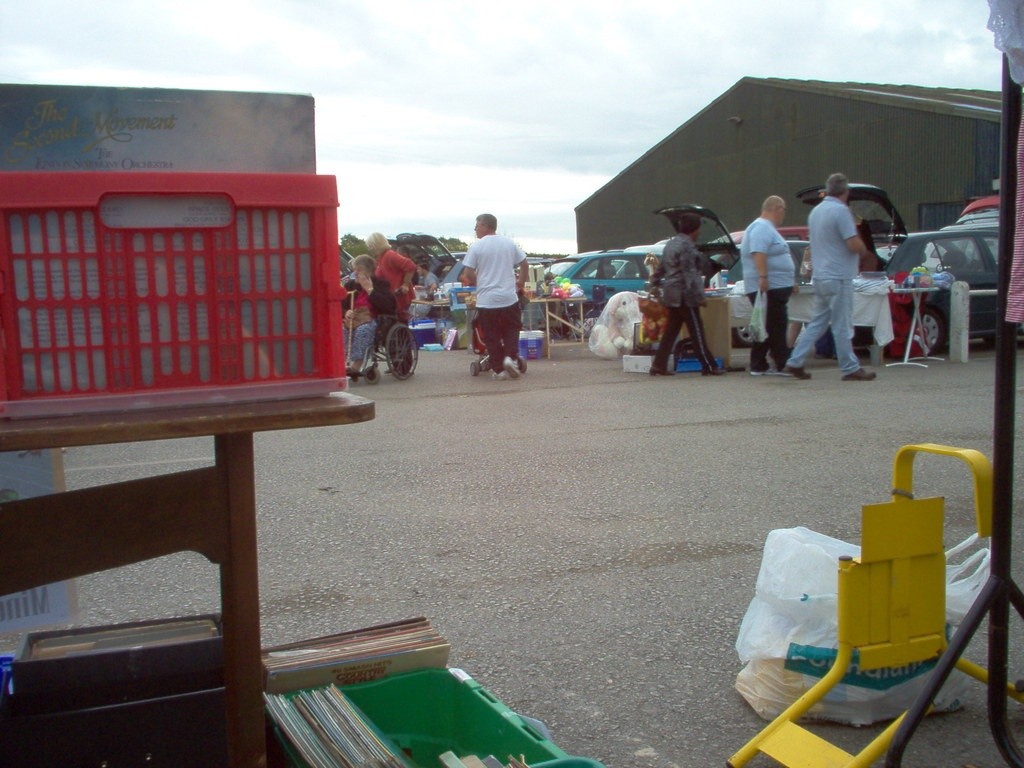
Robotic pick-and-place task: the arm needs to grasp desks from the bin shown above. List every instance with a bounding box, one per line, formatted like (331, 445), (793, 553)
(411, 299), (449, 333)
(681, 294), (753, 371)
(0, 392), (374, 768)
(531, 296), (587, 359)
(748, 278), (895, 366)
(884, 286), (945, 368)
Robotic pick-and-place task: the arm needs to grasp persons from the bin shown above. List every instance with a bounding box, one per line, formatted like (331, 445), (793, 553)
(366, 232), (416, 375)
(785, 173), (877, 381)
(397, 245), (419, 286)
(648, 214), (727, 375)
(341, 258), (355, 286)
(460, 214), (529, 380)
(741, 195), (795, 376)
(414, 262), (439, 290)
(341, 255), (397, 381)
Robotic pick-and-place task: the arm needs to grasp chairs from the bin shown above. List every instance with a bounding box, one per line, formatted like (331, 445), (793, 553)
(610, 265), (616, 278)
(943, 250), (983, 283)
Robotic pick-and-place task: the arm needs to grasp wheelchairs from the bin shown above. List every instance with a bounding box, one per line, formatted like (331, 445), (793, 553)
(346, 288), (419, 385)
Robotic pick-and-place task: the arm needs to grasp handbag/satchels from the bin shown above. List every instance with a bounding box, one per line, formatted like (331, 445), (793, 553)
(749, 288), (769, 342)
(733, 524), (991, 728)
(639, 287), (665, 319)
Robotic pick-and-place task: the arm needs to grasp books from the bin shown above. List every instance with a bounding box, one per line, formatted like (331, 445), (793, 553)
(263, 682), (417, 768)
(255, 616), (450, 694)
(439, 750), (531, 768)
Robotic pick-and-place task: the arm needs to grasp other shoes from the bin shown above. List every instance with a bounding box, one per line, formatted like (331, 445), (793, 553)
(784, 364), (812, 379)
(384, 369), (394, 375)
(751, 362), (774, 376)
(842, 368), (876, 381)
(703, 368), (725, 375)
(346, 367), (359, 381)
(650, 367), (674, 377)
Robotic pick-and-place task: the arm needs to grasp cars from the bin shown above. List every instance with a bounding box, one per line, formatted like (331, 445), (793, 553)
(795, 183), (1024, 356)
(654, 204), (889, 349)
(397, 232), (551, 286)
(544, 250), (663, 336)
(587, 195), (1000, 279)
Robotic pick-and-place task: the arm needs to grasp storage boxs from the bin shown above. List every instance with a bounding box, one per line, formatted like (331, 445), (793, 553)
(408, 322), (436, 350)
(519, 329), (545, 360)
(0, 170), (350, 421)
(623, 354), (674, 373)
(265, 666), (606, 768)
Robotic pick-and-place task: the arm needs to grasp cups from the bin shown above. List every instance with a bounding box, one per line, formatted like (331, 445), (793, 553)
(701, 276), (705, 284)
(465, 300), (476, 310)
(444, 282), (462, 289)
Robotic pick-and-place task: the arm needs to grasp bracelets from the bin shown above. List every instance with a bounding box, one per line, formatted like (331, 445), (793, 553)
(760, 276), (767, 278)
(367, 288), (374, 291)
(404, 281), (411, 284)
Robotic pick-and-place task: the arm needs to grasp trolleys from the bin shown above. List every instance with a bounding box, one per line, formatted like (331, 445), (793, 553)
(470, 286), (527, 377)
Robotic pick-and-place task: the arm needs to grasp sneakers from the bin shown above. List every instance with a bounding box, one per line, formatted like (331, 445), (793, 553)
(493, 371), (507, 379)
(503, 356), (520, 378)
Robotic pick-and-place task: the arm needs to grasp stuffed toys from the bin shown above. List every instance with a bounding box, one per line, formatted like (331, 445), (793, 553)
(644, 252), (658, 276)
(536, 277), (585, 297)
(590, 291), (670, 358)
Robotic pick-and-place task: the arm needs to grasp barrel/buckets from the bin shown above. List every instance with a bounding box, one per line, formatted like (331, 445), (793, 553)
(414, 285), (425, 301)
(408, 317), (460, 350)
(519, 330), (545, 360)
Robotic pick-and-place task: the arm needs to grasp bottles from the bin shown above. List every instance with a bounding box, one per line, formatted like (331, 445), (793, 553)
(721, 270), (728, 287)
(433, 286), (448, 300)
